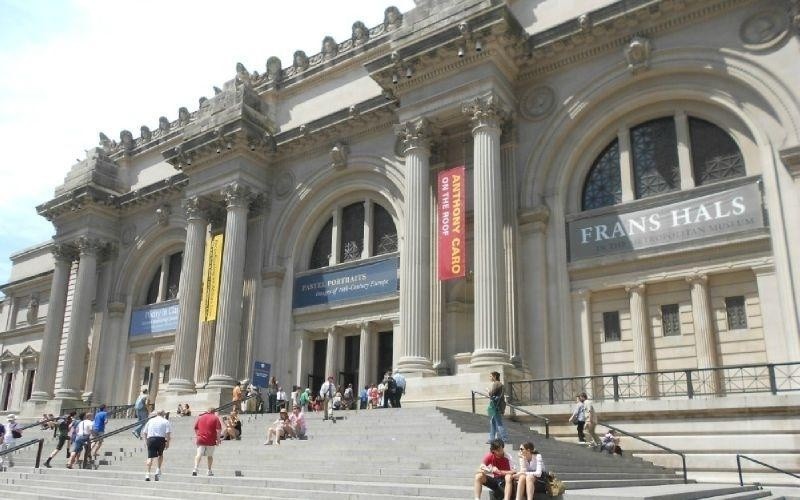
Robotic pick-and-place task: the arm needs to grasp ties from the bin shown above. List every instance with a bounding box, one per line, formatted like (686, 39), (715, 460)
(329, 384), (333, 398)
(280, 393), (282, 400)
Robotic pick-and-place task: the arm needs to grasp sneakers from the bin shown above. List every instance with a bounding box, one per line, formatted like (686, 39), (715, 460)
(131, 430), (139, 439)
(264, 440), (280, 445)
(43, 461), (98, 468)
(145, 471), (215, 481)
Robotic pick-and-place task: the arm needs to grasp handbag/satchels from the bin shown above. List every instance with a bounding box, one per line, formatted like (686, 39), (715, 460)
(11, 428), (22, 439)
(486, 398), (500, 418)
(545, 476), (568, 498)
(572, 418), (580, 426)
(74, 434), (90, 445)
(318, 393), (327, 402)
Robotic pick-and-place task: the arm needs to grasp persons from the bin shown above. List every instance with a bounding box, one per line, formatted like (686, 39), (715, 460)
(39, 404), (108, 469)
(132, 388), (149, 439)
(602, 429), (619, 455)
(222, 412), (242, 441)
(245, 368), (406, 419)
(220, 410), (238, 440)
(177, 404), (184, 415)
(143, 408), (172, 481)
(179, 404), (191, 416)
(611, 429), (623, 457)
(474, 438), (519, 500)
(190, 406), (222, 476)
(1, 414), (23, 469)
(0, 423), (6, 446)
(232, 381), (244, 414)
(282, 406), (307, 440)
(485, 371), (511, 444)
(510, 441), (547, 500)
(568, 396), (586, 443)
(264, 408), (290, 446)
(580, 392), (603, 451)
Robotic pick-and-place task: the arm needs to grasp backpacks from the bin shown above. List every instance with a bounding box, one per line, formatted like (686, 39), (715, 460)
(343, 388), (352, 399)
(133, 394), (147, 411)
(387, 376), (398, 395)
(57, 413), (72, 431)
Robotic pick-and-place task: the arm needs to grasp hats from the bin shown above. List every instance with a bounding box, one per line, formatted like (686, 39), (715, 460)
(305, 388), (313, 394)
(6, 413), (16, 422)
(292, 404), (302, 411)
(279, 407), (288, 415)
